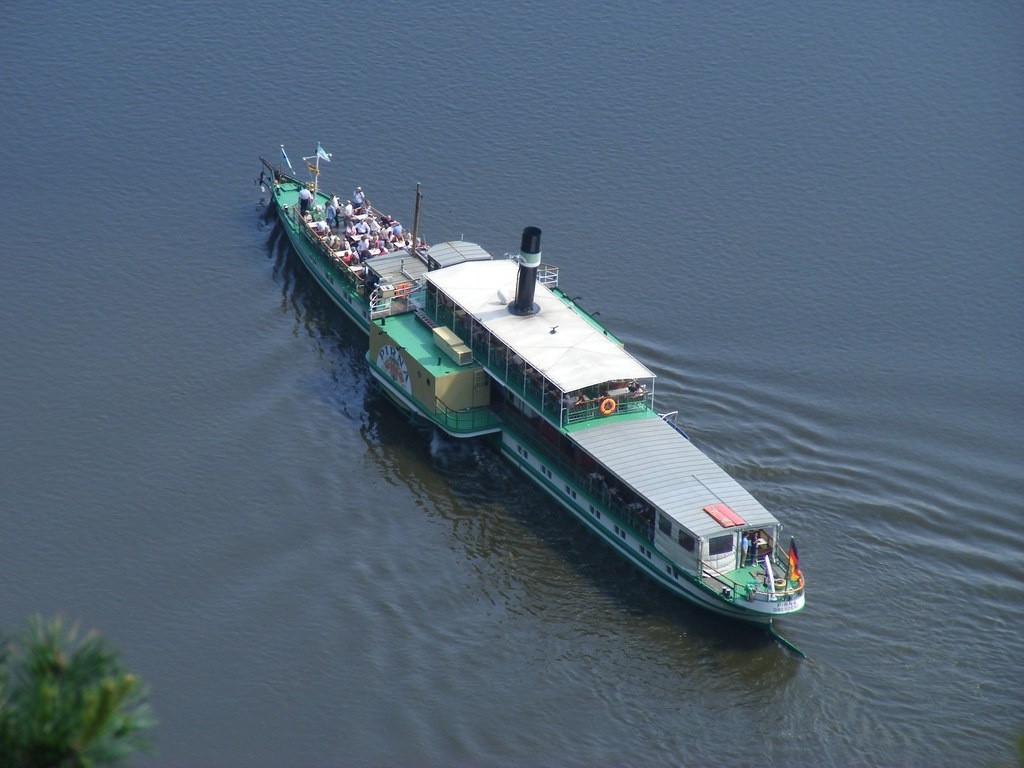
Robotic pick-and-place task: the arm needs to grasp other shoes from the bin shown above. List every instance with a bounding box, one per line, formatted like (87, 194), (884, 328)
(741, 565), (746, 568)
(752, 564), (756, 568)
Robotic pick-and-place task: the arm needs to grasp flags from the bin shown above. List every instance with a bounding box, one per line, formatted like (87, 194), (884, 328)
(315, 145), (330, 162)
(307, 161), (319, 175)
(789, 539), (803, 587)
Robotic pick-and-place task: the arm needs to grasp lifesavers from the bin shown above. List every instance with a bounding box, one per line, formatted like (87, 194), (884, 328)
(600, 398), (616, 415)
(396, 283), (411, 298)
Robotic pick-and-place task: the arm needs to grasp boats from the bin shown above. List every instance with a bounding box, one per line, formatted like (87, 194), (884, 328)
(259, 157), (805, 661)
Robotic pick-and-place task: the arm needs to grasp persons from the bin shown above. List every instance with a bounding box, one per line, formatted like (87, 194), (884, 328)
(304, 187), (426, 290)
(629, 382), (643, 401)
(556, 389), (586, 411)
(750, 531), (760, 567)
(298, 185), (310, 217)
(587, 473), (608, 488)
(741, 532), (749, 567)
(438, 293), (556, 402)
